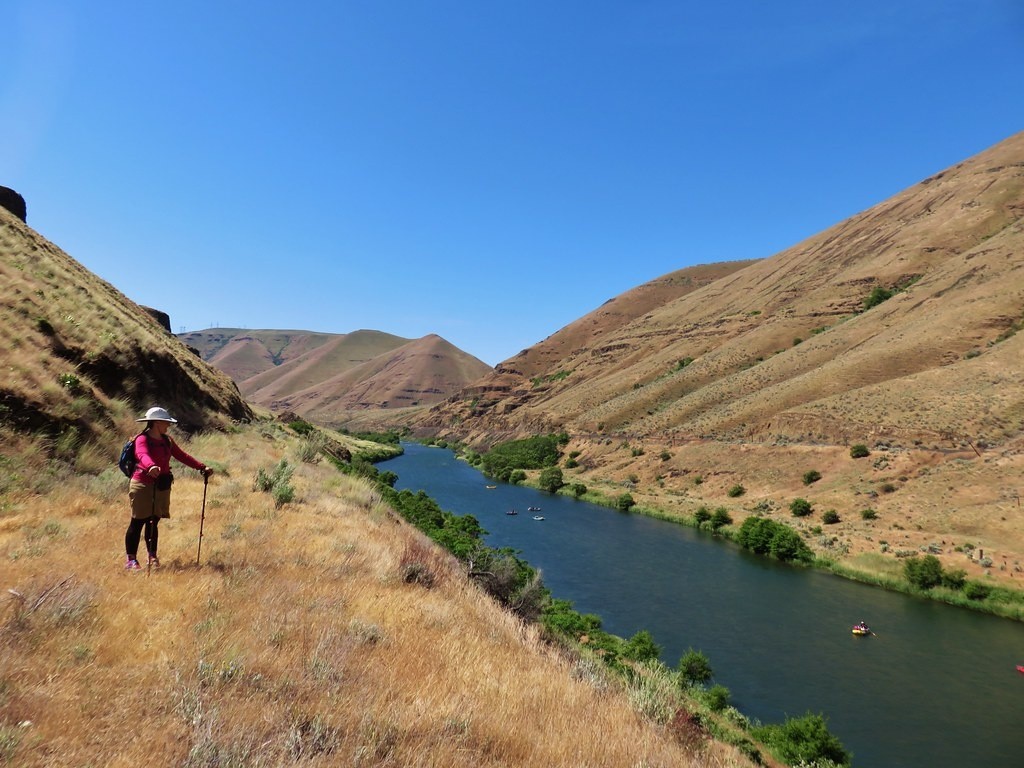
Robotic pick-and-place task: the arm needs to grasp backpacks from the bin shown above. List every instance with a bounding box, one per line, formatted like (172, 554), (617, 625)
(119, 432), (172, 478)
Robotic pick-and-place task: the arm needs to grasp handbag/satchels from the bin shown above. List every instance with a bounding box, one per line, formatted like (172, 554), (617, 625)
(156, 474), (172, 491)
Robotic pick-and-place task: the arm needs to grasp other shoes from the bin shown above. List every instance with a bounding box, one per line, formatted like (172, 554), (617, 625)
(146, 556), (160, 569)
(125, 556), (142, 571)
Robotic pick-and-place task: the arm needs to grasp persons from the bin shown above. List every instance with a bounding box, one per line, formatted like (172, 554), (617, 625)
(861, 622), (868, 629)
(125, 407), (214, 571)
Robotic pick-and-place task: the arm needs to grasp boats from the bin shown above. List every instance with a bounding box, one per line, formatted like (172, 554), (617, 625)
(506, 509), (517, 515)
(528, 507), (541, 511)
(852, 625), (870, 635)
(486, 486), (497, 489)
(532, 516), (546, 521)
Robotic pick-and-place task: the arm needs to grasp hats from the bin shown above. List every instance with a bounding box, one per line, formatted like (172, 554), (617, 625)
(136, 407), (177, 423)
